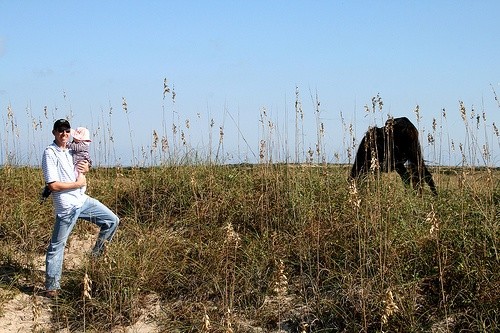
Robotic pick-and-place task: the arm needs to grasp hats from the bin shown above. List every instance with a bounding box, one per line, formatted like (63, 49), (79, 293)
(53, 118), (71, 129)
(70, 126), (92, 142)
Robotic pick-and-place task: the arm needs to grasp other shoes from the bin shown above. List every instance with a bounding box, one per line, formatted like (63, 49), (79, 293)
(47, 290), (57, 298)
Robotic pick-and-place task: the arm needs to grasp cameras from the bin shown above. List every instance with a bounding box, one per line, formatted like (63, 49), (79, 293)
(42, 186), (52, 198)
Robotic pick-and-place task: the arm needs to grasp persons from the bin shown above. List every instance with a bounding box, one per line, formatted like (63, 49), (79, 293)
(42, 118), (117, 300)
(67, 127), (91, 194)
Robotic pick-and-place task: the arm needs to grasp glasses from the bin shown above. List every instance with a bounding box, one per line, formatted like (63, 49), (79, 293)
(54, 126), (71, 133)
